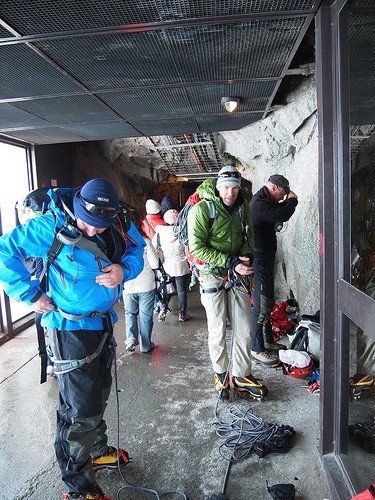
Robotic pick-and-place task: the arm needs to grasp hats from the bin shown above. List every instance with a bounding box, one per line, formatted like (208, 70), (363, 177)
(268, 174), (290, 195)
(163, 209), (179, 225)
(145, 199), (161, 215)
(215, 166), (242, 190)
(73, 178), (121, 228)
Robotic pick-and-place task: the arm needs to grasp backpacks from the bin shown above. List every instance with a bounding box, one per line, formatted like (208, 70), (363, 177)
(12, 185), (129, 295)
(173, 191), (248, 247)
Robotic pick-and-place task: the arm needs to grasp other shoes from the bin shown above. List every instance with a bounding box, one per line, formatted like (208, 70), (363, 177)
(139, 341), (154, 353)
(178, 312), (190, 322)
(157, 308), (166, 323)
(125, 340), (139, 352)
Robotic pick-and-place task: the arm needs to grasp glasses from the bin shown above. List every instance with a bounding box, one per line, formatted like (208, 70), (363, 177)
(79, 197), (120, 220)
(277, 189), (285, 197)
(218, 171), (241, 179)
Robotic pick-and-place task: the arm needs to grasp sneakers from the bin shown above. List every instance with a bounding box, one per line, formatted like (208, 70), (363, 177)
(63, 485), (112, 500)
(263, 342), (287, 351)
(232, 374), (268, 403)
(250, 352), (278, 369)
(213, 371), (231, 402)
(91, 446), (130, 470)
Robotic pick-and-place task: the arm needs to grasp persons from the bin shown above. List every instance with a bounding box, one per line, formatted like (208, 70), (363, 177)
(0, 177), (148, 500)
(248, 174), (298, 368)
(187, 166), (268, 400)
(145, 196), (192, 322)
(121, 212), (160, 353)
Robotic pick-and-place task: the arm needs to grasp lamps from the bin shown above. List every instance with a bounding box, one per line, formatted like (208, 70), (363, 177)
(221, 97), (241, 114)
